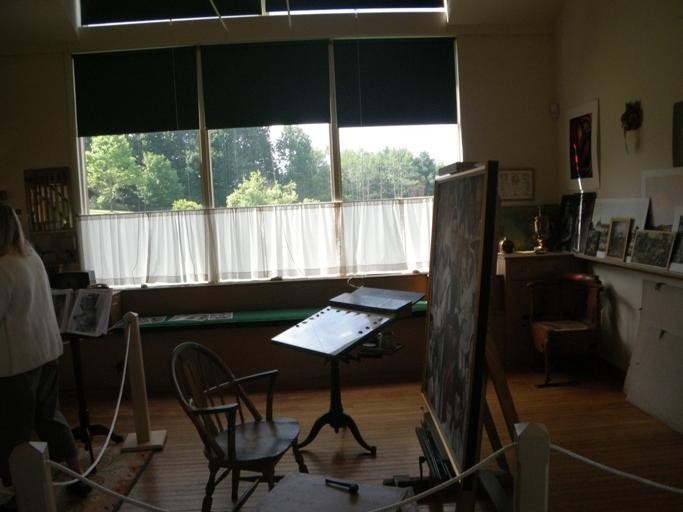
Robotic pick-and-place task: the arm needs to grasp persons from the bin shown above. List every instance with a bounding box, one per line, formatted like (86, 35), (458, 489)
(0, 204), (92, 501)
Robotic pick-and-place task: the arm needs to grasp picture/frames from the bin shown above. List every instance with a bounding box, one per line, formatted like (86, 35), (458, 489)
(630, 229), (676, 270)
(497, 168), (535, 201)
(606, 216), (633, 261)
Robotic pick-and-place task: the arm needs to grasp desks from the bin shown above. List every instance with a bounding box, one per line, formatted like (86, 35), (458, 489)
(249, 471), (410, 511)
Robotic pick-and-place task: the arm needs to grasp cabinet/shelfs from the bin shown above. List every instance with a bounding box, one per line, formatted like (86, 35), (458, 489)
(497, 249), (571, 340)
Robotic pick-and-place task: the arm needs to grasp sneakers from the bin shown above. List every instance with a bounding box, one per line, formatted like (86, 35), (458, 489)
(65, 481), (95, 496)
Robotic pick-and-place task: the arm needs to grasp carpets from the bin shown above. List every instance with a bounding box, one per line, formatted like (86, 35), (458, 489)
(2, 428), (155, 511)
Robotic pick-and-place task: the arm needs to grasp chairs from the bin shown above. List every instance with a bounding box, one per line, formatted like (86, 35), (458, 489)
(525, 273), (601, 387)
(170, 341), (308, 512)
